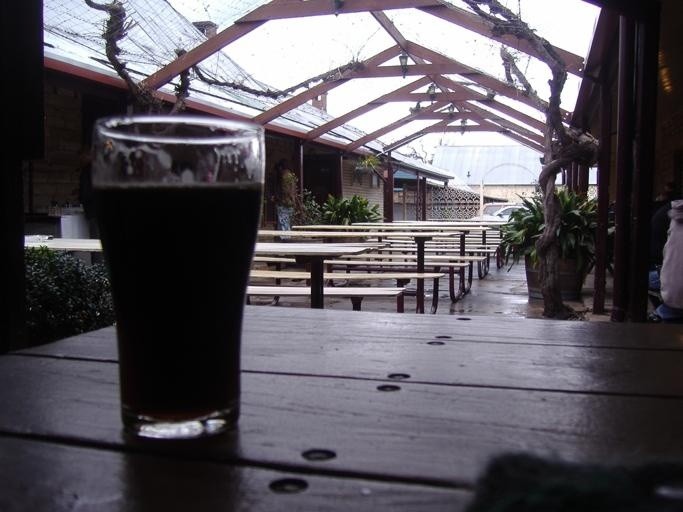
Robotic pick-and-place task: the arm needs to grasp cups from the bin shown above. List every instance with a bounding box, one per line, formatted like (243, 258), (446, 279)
(88, 113), (268, 444)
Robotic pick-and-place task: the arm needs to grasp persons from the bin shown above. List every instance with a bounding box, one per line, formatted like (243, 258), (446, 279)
(273, 158), (297, 243)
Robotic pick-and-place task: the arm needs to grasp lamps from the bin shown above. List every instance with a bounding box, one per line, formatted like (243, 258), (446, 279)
(396, 55), (465, 131)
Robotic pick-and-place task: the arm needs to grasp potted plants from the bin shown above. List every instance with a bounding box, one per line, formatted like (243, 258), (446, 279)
(498, 189), (612, 300)
(318, 196), (381, 242)
(276, 172), (299, 238)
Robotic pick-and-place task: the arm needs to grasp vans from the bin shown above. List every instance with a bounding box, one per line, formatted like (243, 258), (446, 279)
(480, 201), (521, 219)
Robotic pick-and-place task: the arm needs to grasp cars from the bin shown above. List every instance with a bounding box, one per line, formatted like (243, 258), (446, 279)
(471, 205), (528, 222)
